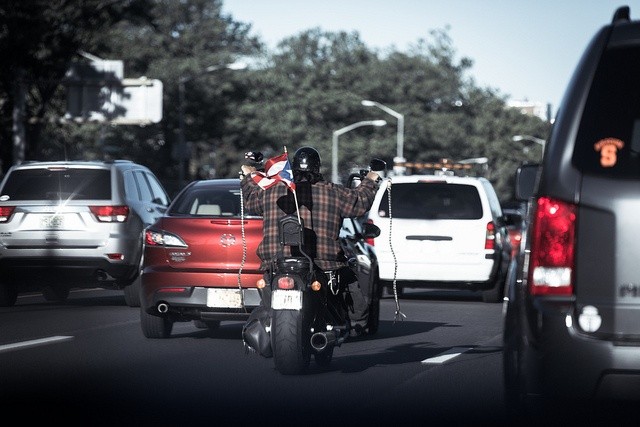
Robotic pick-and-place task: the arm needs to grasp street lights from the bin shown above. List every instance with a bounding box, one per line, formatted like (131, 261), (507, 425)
(511, 134), (545, 160)
(361, 100), (406, 157)
(177, 56), (249, 184)
(332, 118), (388, 186)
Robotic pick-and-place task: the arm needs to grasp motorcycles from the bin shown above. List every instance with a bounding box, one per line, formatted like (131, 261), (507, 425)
(240, 151), (390, 376)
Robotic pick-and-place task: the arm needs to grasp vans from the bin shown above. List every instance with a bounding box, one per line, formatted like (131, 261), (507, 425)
(341, 175), (511, 301)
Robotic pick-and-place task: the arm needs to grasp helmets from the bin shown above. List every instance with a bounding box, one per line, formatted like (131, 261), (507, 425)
(293, 146), (321, 172)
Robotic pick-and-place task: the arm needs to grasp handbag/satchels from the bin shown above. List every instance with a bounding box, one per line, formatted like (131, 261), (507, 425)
(338, 238), (379, 297)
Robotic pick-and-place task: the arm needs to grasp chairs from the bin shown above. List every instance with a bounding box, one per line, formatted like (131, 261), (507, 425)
(195, 203), (221, 215)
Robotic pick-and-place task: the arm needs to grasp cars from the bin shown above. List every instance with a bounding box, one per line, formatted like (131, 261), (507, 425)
(501, 209), (525, 273)
(139, 178), (381, 339)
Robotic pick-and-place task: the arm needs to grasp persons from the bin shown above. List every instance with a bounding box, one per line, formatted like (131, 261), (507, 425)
(239, 145), (383, 336)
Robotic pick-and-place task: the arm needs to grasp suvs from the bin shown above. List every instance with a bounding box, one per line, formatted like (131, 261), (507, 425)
(502, 3), (640, 424)
(0, 156), (173, 308)
